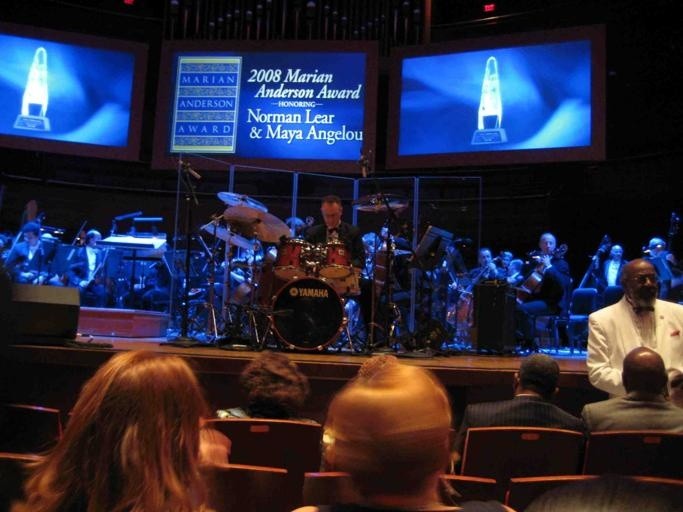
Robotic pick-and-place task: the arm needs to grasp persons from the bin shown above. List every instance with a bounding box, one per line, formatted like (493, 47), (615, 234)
(456, 353), (585, 472)
(587, 259), (683, 409)
(289, 355), (518, 512)
(581, 347), (683, 432)
(211, 350), (319, 424)
(3, 195), (676, 355)
(11, 349), (228, 511)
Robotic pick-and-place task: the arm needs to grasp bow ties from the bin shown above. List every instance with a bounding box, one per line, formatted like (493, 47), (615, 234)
(633, 305), (655, 315)
(328, 226), (340, 234)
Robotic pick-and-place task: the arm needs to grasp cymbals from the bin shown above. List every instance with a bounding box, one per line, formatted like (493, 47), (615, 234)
(205, 225), (251, 249)
(352, 193), (409, 212)
(218, 192), (268, 213)
(224, 208), (291, 242)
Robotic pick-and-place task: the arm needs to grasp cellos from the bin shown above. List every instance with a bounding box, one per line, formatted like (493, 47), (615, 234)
(515, 244), (568, 303)
(450, 258), (499, 347)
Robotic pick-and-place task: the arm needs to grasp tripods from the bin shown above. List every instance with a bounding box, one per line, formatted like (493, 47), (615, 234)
(339, 212), (451, 351)
(177, 214), (260, 343)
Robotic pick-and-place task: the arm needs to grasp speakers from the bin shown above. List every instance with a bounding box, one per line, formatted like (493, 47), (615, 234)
(475, 283), (518, 353)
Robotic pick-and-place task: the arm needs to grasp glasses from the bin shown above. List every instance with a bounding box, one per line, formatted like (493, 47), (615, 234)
(624, 274), (659, 284)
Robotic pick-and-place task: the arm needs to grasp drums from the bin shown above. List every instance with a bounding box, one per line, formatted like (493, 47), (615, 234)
(321, 266), (361, 297)
(319, 241), (352, 278)
(271, 238), (318, 281)
(271, 277), (346, 350)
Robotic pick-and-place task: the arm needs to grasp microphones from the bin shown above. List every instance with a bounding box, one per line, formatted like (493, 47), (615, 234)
(177, 157), (201, 179)
(360, 155), (367, 179)
